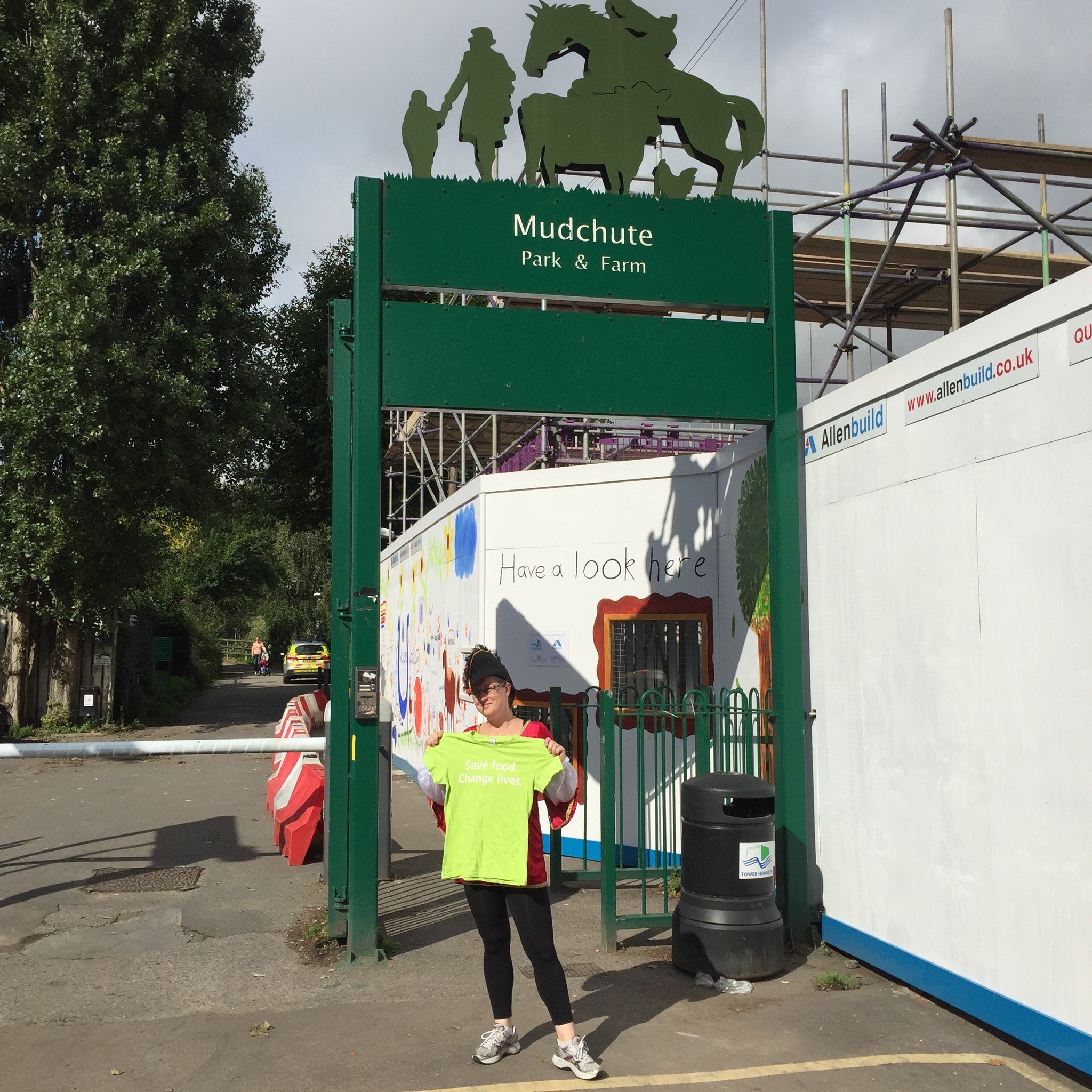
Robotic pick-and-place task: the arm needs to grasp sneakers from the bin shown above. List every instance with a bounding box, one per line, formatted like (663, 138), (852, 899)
(551, 1037), (604, 1080)
(470, 1023), (521, 1066)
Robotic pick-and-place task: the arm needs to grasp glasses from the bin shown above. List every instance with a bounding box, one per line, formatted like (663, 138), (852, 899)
(468, 677), (510, 698)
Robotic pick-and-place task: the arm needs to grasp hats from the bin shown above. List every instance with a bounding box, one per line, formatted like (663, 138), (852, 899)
(460, 643), (515, 686)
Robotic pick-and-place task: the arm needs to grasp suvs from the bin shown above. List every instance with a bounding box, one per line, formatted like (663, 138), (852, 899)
(283, 639), (331, 684)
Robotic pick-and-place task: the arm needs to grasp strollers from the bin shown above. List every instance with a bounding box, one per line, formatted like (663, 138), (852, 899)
(258, 653), (270, 676)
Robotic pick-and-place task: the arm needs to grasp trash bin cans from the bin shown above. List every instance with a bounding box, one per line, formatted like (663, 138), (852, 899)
(79, 686), (100, 718)
(670, 771), (786, 981)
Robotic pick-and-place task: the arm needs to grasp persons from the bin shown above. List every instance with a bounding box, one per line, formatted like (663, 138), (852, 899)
(251, 636), (266, 676)
(258, 655), (269, 675)
(419, 645), (605, 1083)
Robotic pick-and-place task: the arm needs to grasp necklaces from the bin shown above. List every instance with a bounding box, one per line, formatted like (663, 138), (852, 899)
(486, 715), (514, 737)
